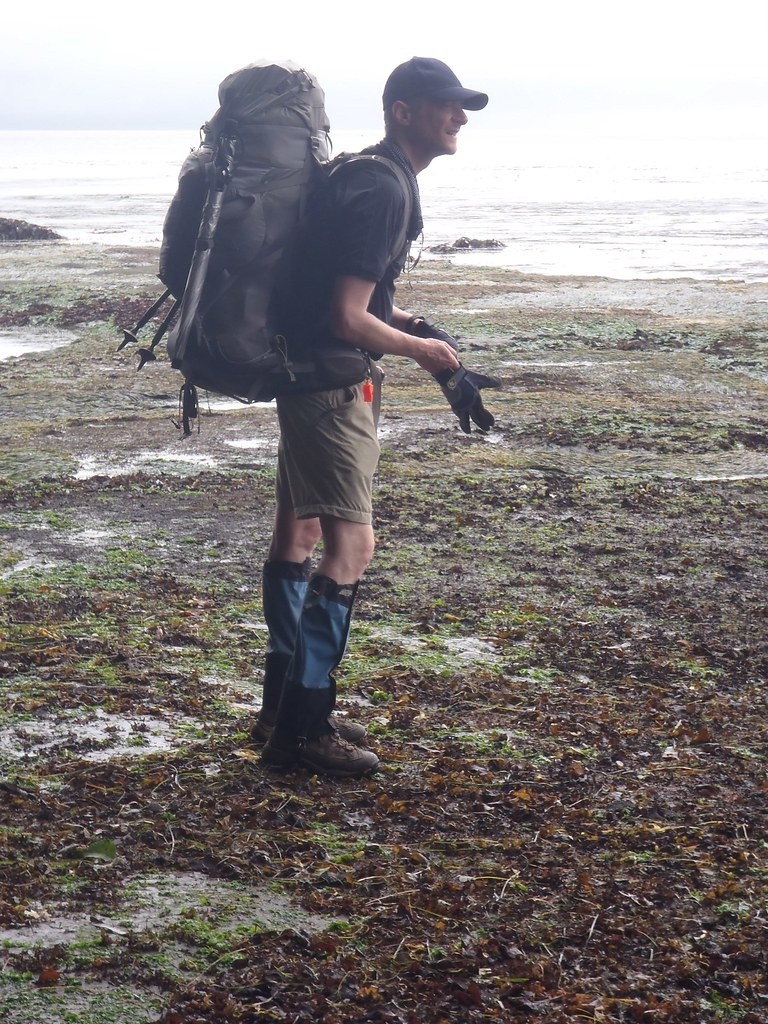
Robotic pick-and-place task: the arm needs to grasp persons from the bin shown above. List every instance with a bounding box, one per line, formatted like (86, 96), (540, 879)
(248, 56), (489, 777)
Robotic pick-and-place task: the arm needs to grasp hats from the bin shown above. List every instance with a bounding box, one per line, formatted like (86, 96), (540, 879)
(383, 56), (488, 111)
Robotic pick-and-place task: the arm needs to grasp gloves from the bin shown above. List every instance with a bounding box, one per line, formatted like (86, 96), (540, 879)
(431, 361), (502, 434)
(411, 321), (458, 358)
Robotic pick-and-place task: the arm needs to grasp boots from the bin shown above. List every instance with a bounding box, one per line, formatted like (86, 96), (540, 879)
(251, 556), (368, 745)
(261, 572), (380, 777)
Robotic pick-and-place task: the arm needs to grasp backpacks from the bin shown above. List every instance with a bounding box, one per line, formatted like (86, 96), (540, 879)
(115, 64), (413, 440)
